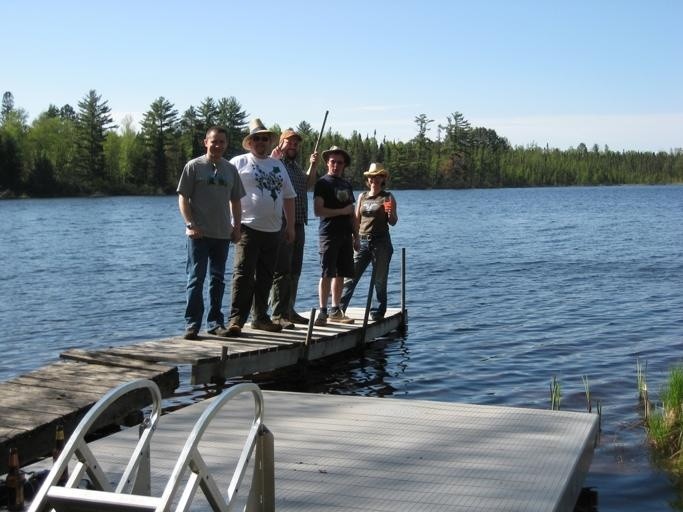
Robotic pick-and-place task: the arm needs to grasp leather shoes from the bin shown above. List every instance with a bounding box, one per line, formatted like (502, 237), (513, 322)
(370, 313), (384, 320)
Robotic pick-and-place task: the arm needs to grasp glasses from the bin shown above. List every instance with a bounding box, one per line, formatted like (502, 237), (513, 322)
(212, 161), (217, 177)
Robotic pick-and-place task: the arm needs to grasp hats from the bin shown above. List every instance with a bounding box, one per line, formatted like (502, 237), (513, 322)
(242, 118), (278, 151)
(363, 163), (387, 176)
(321, 145), (350, 166)
(279, 129), (301, 142)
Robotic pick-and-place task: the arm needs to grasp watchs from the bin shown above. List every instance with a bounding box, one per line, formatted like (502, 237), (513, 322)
(184, 219), (196, 229)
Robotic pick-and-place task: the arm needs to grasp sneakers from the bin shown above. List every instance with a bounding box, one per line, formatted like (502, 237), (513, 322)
(184, 311), (309, 339)
(314, 312), (328, 325)
(328, 310), (353, 324)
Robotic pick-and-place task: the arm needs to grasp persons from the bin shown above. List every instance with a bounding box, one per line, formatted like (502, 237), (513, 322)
(312, 144), (356, 326)
(326, 163), (399, 320)
(175, 124), (247, 339)
(267, 130), (319, 327)
(215, 116), (298, 332)
